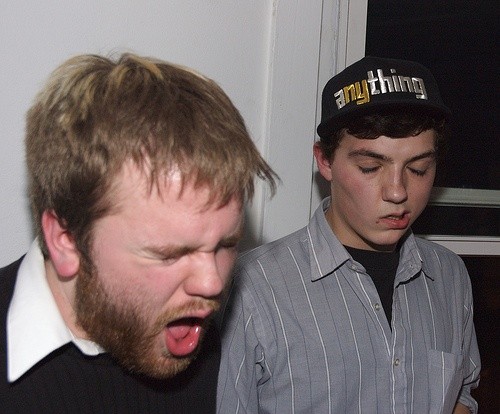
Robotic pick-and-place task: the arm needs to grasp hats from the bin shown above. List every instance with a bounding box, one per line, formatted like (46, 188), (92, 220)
(316, 54), (452, 138)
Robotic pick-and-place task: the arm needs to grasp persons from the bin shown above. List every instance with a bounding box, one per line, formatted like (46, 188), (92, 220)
(212, 54), (486, 414)
(0, 49), (285, 414)
